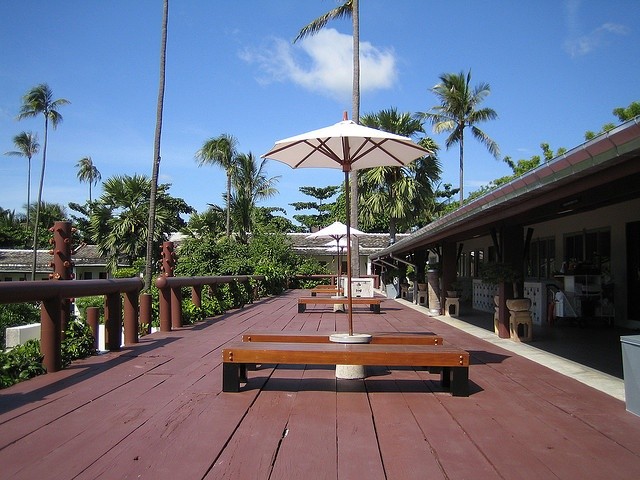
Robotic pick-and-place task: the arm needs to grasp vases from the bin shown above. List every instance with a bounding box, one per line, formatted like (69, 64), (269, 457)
(418, 284), (427, 291)
(447, 290), (457, 297)
(494, 295), (500, 307)
(506, 298), (532, 311)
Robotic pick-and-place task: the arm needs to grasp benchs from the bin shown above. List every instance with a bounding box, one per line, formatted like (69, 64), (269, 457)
(298, 296), (382, 314)
(222, 340), (469, 398)
(242, 328), (443, 374)
(315, 285), (336, 288)
(310, 288), (345, 297)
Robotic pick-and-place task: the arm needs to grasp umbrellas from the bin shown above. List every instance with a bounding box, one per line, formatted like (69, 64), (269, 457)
(325, 239), (363, 289)
(326, 246), (348, 281)
(261, 112), (435, 335)
(305, 216), (367, 297)
